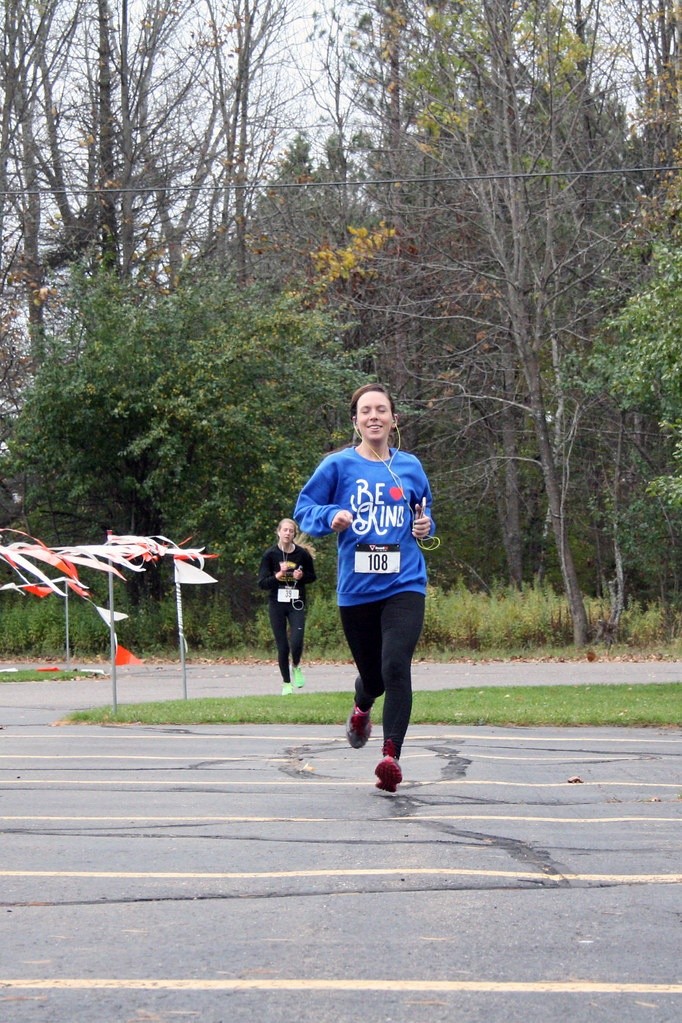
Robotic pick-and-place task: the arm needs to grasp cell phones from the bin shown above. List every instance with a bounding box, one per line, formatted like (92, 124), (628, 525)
(418, 497), (426, 518)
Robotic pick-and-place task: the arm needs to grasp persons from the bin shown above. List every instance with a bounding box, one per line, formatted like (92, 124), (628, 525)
(292, 382), (436, 793)
(257, 518), (317, 696)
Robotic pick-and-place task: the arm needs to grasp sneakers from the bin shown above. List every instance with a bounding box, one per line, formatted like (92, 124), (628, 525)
(292, 665), (305, 688)
(375, 754), (402, 793)
(345, 705), (371, 749)
(282, 682), (292, 695)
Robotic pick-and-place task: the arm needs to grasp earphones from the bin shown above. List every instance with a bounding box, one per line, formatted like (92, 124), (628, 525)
(278, 531), (280, 536)
(352, 416), (356, 422)
(393, 413), (398, 419)
